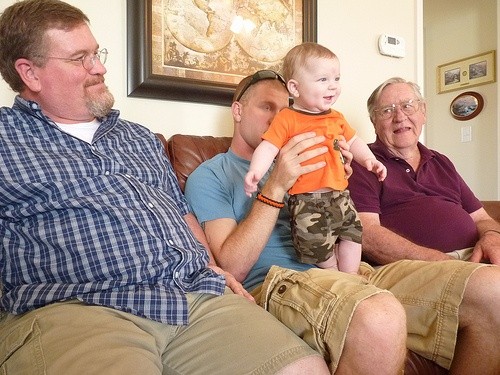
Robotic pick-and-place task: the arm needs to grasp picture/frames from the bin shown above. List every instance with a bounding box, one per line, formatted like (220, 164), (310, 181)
(126, 0), (318, 107)
(450, 91), (484, 121)
(437, 50), (497, 95)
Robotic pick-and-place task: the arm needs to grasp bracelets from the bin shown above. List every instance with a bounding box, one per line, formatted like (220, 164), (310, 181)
(257, 192), (285, 209)
(482, 230), (500, 234)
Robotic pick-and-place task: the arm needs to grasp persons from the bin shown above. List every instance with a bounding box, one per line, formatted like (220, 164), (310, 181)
(0, 0), (331, 375)
(183, 70), (500, 375)
(244, 42), (387, 276)
(346, 77), (500, 264)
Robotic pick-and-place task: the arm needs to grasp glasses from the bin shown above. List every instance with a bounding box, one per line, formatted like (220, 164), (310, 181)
(373, 99), (423, 121)
(33, 47), (109, 70)
(236, 69), (291, 104)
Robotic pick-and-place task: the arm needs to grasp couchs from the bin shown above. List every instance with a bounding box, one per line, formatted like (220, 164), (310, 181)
(155, 133), (500, 375)
